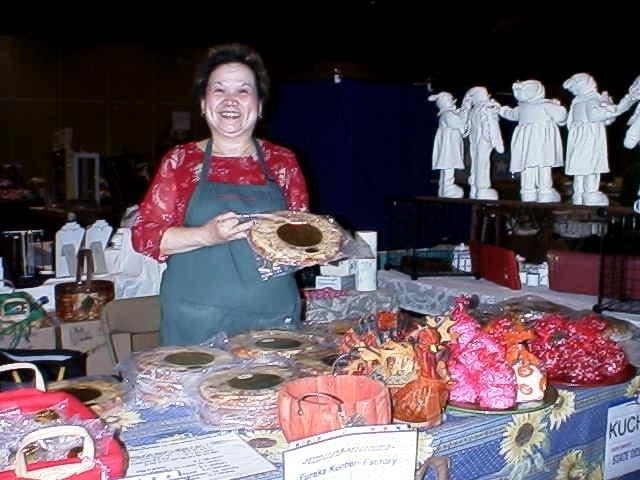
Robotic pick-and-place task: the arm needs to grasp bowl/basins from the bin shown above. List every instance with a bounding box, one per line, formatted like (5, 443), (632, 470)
(277, 352), (393, 443)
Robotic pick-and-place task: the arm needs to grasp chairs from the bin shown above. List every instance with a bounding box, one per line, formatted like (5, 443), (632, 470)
(1, 220), (166, 312)
(101, 295), (162, 378)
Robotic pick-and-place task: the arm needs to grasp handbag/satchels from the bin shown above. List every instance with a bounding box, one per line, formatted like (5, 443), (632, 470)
(0, 279), (48, 334)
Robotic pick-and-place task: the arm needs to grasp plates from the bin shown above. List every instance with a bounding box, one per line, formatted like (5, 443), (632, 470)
(445, 384), (558, 415)
(547, 364), (637, 388)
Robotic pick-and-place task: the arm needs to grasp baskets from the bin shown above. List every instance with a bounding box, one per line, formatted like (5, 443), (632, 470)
(55, 250), (115, 322)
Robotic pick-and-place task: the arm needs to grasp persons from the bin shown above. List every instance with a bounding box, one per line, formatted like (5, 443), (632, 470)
(130, 43), (310, 348)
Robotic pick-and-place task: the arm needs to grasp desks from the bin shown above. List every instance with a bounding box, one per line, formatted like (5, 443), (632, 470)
(0, 294), (640, 480)
(376, 267), (639, 366)
